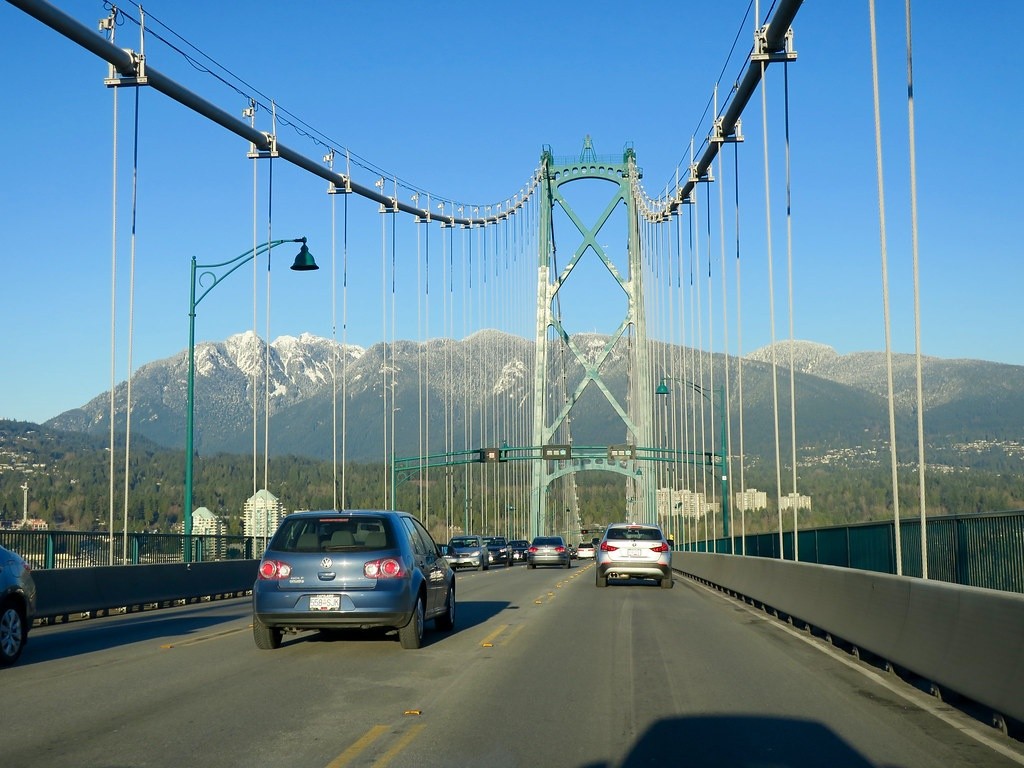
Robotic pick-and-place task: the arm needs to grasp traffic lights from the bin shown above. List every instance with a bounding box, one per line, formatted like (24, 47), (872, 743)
(581, 530), (588, 534)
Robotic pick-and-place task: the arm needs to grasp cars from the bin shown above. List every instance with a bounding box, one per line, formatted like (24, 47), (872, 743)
(443, 536), (490, 571)
(578, 541), (596, 559)
(567, 547), (578, 558)
(0, 544), (37, 666)
(482, 537), (513, 567)
(251, 508), (457, 648)
(527, 536), (572, 569)
(506, 540), (532, 564)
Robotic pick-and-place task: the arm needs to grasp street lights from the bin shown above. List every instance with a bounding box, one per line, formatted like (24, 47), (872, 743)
(463, 439), (508, 535)
(635, 466), (656, 525)
(655, 376), (730, 538)
(178, 237), (318, 561)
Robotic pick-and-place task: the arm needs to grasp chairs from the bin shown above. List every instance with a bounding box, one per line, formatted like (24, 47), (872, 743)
(365, 532), (386, 547)
(614, 534), (626, 538)
(297, 533), (319, 548)
(330, 531), (355, 546)
(639, 535), (653, 539)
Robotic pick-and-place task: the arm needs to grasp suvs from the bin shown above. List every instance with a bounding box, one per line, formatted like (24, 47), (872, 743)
(592, 522), (675, 589)
